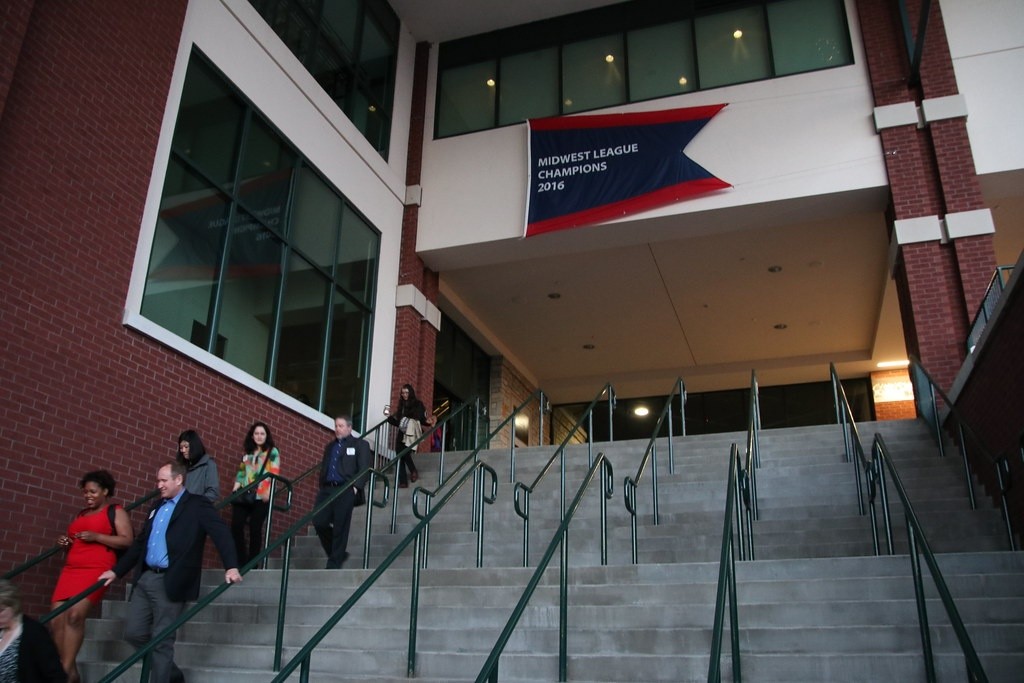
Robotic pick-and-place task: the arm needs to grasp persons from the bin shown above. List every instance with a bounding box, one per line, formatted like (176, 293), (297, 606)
(0, 579), (66, 683)
(383, 383), (433, 488)
(431, 414), (441, 453)
(98, 461), (243, 683)
(229, 421), (280, 569)
(50, 469), (134, 683)
(177, 429), (220, 502)
(311, 415), (372, 570)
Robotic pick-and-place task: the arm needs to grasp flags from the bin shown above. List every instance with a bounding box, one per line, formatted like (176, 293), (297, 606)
(521, 102), (733, 241)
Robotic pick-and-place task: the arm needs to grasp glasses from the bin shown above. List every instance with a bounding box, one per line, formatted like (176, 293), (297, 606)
(401, 393), (409, 396)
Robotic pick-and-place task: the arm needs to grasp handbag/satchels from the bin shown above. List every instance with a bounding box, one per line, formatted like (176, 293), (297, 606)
(354, 488), (366, 507)
(107, 504), (129, 566)
(230, 489), (256, 506)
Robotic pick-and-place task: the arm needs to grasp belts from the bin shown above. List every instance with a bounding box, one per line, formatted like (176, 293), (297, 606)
(322, 481), (348, 488)
(144, 563), (169, 572)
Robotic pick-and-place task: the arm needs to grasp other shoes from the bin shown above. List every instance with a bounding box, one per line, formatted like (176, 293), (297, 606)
(400, 482), (408, 488)
(326, 552), (349, 569)
(411, 470), (418, 482)
